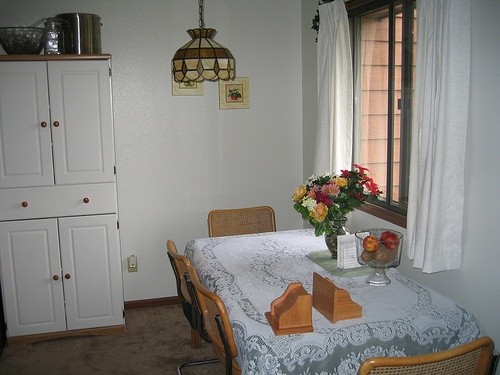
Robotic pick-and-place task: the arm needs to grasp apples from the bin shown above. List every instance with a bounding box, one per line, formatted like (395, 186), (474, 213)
(362, 231), (400, 264)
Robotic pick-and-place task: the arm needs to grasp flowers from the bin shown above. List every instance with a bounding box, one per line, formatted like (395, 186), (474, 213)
(293, 163), (384, 229)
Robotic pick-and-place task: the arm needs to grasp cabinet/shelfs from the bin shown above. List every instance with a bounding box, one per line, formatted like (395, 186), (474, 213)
(0, 53), (129, 347)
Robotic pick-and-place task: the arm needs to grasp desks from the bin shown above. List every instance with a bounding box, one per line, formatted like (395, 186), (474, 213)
(188, 227), (475, 375)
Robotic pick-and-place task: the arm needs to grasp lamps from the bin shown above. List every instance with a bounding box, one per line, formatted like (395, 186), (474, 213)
(170, 0), (237, 84)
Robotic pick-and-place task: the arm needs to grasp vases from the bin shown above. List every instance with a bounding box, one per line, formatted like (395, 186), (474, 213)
(324, 218), (350, 260)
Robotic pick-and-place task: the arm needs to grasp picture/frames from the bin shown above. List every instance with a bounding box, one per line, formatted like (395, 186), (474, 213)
(172, 75), (204, 96)
(219, 78), (250, 109)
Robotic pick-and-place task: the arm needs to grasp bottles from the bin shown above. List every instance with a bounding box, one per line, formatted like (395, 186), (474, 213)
(43, 17), (64, 53)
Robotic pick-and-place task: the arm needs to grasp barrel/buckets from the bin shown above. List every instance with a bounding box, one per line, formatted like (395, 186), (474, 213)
(57, 14), (102, 54)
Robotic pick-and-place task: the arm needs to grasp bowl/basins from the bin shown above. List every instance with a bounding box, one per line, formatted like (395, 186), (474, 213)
(0, 27), (48, 54)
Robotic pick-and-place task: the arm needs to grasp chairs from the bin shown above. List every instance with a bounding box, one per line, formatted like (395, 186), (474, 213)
(166, 206), (500, 375)
